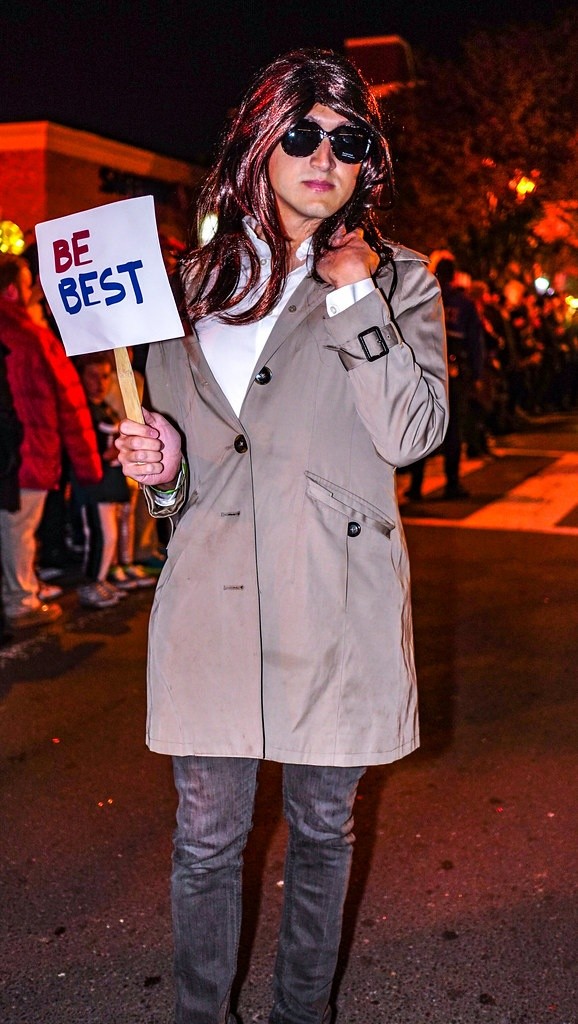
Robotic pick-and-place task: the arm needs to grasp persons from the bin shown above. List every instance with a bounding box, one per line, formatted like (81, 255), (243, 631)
(1, 234), (502, 630)
(115, 47), (449, 1024)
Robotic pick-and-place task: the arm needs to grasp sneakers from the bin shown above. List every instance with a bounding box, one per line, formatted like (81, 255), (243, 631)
(98, 580), (128, 597)
(78, 582), (120, 607)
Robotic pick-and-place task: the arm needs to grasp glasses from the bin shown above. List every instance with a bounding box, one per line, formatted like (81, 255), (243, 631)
(280, 120), (377, 164)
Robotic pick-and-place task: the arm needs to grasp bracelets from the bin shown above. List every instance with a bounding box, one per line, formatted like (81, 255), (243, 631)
(149, 455), (187, 494)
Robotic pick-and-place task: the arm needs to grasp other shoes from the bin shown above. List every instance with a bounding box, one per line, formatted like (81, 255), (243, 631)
(404, 489), (422, 500)
(125, 562), (158, 586)
(108, 563), (138, 588)
(38, 581), (63, 601)
(8, 601), (63, 629)
(445, 486), (469, 498)
